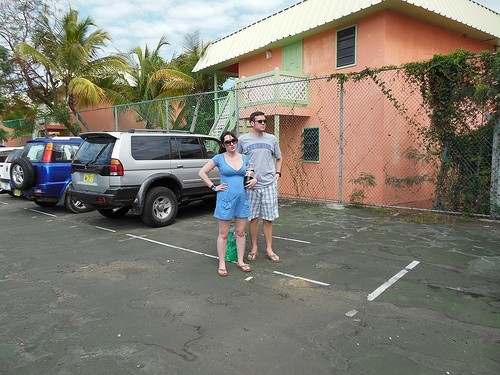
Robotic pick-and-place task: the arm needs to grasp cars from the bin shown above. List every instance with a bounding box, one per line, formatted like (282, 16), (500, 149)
(0, 147), (24, 193)
(0, 147), (12, 164)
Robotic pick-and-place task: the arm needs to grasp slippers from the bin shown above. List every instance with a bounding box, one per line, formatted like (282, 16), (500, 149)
(265, 250), (282, 263)
(245, 250), (259, 261)
(218, 269), (228, 276)
(237, 262), (251, 272)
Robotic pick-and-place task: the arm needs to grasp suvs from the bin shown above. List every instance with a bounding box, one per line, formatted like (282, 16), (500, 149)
(71, 130), (226, 225)
(9, 137), (94, 213)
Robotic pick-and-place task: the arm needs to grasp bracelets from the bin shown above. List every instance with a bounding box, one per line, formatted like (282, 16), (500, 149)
(210, 184), (216, 190)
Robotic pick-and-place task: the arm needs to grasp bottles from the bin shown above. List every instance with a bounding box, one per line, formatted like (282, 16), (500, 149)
(244, 163), (254, 186)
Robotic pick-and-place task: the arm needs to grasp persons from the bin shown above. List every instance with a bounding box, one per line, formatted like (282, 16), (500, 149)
(236, 111), (283, 263)
(255, 177), (259, 182)
(198, 131), (258, 277)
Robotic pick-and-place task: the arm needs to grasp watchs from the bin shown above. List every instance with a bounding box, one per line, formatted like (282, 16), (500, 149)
(212, 185), (217, 190)
(275, 172), (281, 177)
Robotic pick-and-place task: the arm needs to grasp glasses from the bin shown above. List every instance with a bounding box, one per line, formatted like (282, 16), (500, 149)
(223, 138), (238, 146)
(254, 120), (267, 123)
